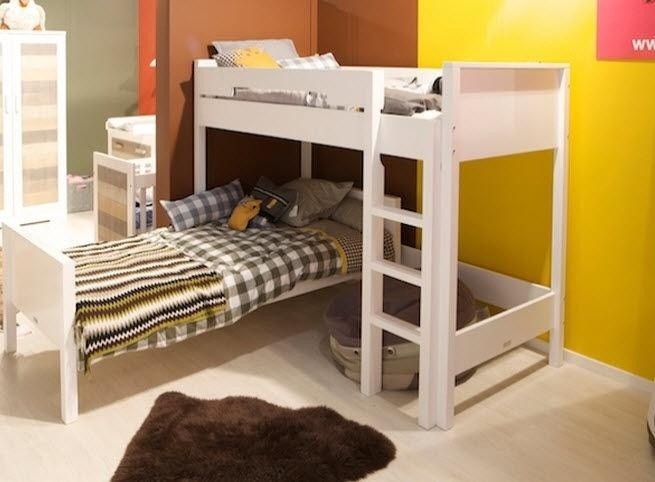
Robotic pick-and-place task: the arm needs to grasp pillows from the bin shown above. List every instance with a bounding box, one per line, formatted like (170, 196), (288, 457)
(159, 177), (354, 232)
(211, 38), (340, 68)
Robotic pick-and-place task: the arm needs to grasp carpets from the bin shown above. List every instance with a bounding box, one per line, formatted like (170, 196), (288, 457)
(109, 392), (396, 482)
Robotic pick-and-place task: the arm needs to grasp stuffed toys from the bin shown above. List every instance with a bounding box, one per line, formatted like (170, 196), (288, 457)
(227, 193), (264, 231)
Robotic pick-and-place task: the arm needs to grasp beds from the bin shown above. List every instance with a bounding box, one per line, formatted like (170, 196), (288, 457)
(194, 58), (571, 160)
(2, 182), (402, 425)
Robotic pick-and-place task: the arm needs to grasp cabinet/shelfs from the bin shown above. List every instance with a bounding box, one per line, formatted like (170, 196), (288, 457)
(93, 116), (156, 243)
(0, 30), (67, 227)
